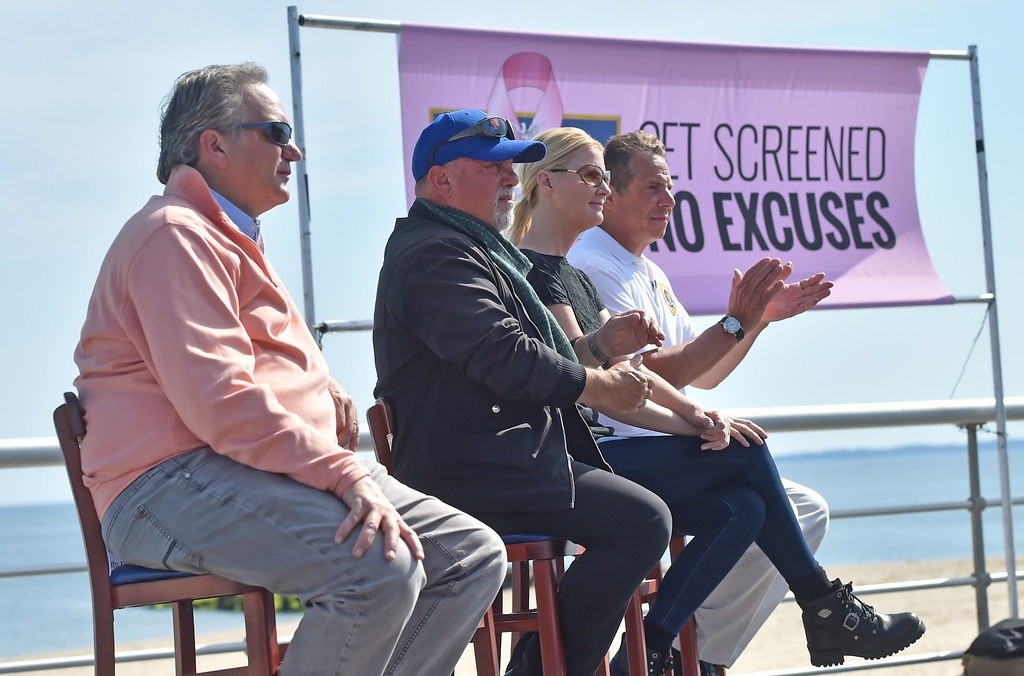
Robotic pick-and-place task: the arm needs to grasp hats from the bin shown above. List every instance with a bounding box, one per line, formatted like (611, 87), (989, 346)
(412, 109), (546, 182)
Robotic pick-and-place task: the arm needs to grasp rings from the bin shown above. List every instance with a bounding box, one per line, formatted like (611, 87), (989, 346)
(354, 421), (357, 424)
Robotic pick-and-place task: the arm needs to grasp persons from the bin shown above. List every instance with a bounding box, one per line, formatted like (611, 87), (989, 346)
(74, 61), (507, 676)
(565, 129), (834, 676)
(373, 108), (671, 676)
(507, 126), (926, 676)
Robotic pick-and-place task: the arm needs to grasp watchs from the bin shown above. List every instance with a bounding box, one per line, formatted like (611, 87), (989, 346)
(718, 316), (744, 342)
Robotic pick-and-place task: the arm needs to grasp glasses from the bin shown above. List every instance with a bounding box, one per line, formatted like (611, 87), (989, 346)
(429, 116), (516, 169)
(550, 164), (611, 187)
(236, 121), (292, 146)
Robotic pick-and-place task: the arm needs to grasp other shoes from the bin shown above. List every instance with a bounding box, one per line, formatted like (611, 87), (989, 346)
(670, 646), (717, 676)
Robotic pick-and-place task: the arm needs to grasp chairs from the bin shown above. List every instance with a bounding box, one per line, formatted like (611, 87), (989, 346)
(510, 534), (702, 676)
(51, 391), (291, 675)
(366, 396), (612, 675)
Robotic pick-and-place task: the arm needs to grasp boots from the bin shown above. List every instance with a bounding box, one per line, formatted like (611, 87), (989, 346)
(609, 632), (670, 676)
(801, 578), (926, 667)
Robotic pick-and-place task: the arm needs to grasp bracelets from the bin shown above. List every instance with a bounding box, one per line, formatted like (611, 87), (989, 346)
(588, 331), (610, 362)
